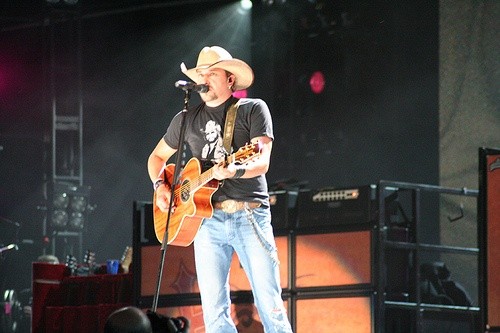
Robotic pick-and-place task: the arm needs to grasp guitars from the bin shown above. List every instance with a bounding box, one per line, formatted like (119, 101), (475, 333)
(152, 140), (263, 247)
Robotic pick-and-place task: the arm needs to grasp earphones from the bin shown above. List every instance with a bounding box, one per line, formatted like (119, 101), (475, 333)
(228, 77), (232, 83)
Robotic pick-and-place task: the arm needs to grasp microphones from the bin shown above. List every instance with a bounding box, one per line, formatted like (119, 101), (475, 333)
(175, 80), (209, 93)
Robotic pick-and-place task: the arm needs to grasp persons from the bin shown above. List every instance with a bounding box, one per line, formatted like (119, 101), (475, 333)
(233, 302), (265, 333)
(147, 46), (294, 333)
(202, 119), (228, 164)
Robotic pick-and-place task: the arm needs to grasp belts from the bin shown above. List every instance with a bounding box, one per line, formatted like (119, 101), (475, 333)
(212, 198), (263, 213)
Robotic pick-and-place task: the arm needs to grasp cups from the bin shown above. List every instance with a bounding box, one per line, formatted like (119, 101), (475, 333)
(106, 259), (119, 275)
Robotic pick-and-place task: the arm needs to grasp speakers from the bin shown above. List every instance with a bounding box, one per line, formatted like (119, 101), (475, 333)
(136, 225), (409, 333)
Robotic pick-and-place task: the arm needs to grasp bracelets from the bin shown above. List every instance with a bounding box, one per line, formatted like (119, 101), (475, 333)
(154, 179), (164, 190)
(233, 164), (246, 179)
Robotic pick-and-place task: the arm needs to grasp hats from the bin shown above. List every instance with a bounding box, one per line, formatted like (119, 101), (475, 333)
(180, 45), (255, 91)
